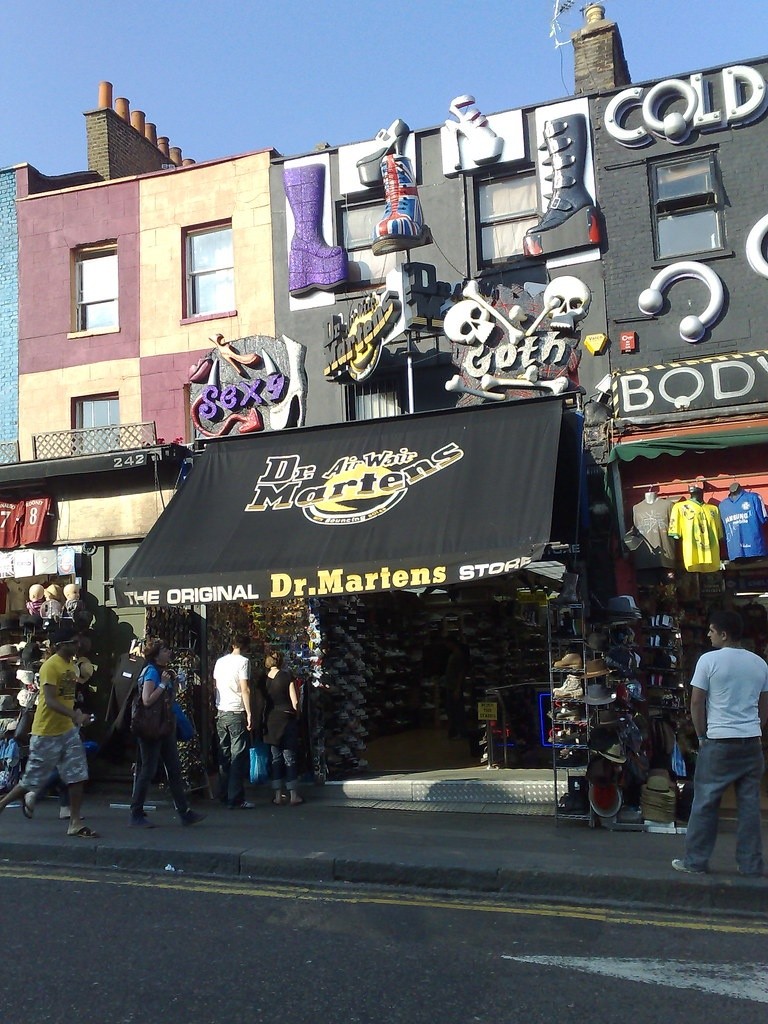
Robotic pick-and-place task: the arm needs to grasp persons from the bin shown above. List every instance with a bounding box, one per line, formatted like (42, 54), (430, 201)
(672, 609), (768, 877)
(26, 584), (44, 616)
(129, 640), (207, 829)
(63, 584), (84, 620)
(22, 774), (85, 821)
(720, 483), (767, 561)
(41, 584), (63, 620)
(256, 651), (304, 806)
(632, 492), (676, 569)
(0, 627), (100, 839)
(669, 486), (724, 573)
(213, 636), (255, 809)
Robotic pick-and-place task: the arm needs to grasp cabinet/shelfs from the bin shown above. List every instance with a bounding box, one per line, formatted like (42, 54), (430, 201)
(0, 610), (79, 808)
(547, 599), (610, 827)
(358, 590), (557, 736)
(640, 623), (711, 723)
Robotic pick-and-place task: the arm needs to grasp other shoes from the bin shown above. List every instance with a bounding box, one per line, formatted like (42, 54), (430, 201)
(672, 859), (705, 874)
(738, 866), (760, 877)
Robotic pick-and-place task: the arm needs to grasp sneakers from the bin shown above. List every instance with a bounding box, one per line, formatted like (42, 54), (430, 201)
(324, 587), (584, 770)
(128, 815), (160, 828)
(180, 808), (210, 825)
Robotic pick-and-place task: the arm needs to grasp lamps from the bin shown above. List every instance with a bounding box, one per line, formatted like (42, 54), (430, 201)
(595, 373), (612, 405)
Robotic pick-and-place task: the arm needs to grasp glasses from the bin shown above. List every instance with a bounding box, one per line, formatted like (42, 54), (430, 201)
(145, 599), (325, 788)
(56, 640), (78, 645)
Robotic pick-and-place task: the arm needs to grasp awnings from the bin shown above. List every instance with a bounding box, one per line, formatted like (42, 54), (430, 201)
(113, 391), (585, 606)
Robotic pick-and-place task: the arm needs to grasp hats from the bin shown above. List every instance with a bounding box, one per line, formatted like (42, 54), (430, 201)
(580, 594), (692, 825)
(0, 583), (96, 794)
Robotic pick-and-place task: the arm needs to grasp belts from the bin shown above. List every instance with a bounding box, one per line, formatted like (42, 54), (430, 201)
(708, 736), (762, 744)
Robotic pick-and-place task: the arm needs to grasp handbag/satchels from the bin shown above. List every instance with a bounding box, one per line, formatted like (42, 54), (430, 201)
(14, 691), (41, 744)
(131, 663), (172, 738)
(250, 746), (269, 784)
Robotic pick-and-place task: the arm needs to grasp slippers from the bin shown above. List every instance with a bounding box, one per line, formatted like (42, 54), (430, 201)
(59, 815), (85, 821)
(227, 801), (253, 809)
(22, 794), (33, 818)
(290, 796), (304, 805)
(67, 827), (97, 838)
(272, 797), (280, 805)
